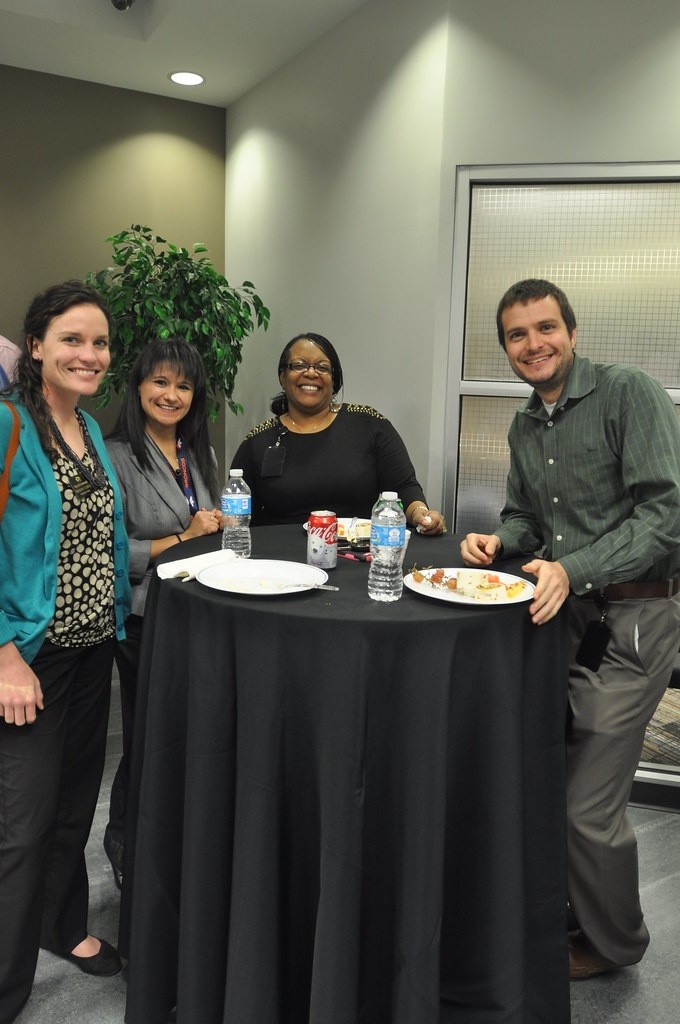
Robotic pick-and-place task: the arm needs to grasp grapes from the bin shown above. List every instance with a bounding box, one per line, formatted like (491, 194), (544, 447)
(414, 569), (458, 589)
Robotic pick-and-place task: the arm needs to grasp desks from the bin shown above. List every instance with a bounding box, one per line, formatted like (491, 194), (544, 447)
(103, 523), (582, 1024)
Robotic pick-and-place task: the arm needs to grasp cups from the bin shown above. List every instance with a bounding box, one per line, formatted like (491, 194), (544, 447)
(398, 530), (411, 567)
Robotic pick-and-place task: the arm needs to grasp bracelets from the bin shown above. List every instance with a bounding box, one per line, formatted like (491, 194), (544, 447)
(411, 506), (428, 522)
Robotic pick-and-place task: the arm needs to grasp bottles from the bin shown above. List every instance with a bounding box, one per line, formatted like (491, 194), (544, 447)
(367, 491), (406, 602)
(221, 469), (251, 558)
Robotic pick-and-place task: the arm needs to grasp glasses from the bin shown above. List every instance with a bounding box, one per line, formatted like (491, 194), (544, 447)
(282, 358), (336, 375)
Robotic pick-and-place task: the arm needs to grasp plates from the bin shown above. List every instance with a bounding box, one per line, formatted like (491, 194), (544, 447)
(302, 518), (371, 540)
(195, 559), (329, 596)
(404, 568), (536, 605)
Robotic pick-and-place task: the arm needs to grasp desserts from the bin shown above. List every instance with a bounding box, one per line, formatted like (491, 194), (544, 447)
(355, 522), (372, 538)
(474, 582), (509, 602)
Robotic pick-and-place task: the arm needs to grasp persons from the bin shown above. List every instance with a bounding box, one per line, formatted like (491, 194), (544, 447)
(101, 339), (239, 889)
(0, 278), (122, 1023)
(229, 331), (447, 537)
(460, 280), (680, 980)
(0, 334), (23, 392)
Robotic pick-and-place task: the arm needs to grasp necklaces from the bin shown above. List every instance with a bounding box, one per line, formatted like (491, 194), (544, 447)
(49, 406), (106, 490)
(286, 410), (332, 433)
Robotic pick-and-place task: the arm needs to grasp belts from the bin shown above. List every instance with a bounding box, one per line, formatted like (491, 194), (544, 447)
(570, 576), (679, 598)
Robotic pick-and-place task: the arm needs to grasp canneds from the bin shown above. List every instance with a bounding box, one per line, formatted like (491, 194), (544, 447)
(307, 510), (338, 570)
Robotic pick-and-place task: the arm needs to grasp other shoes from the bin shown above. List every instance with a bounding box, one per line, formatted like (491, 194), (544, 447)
(568, 931), (642, 980)
(63, 933), (122, 976)
(567, 900), (581, 933)
